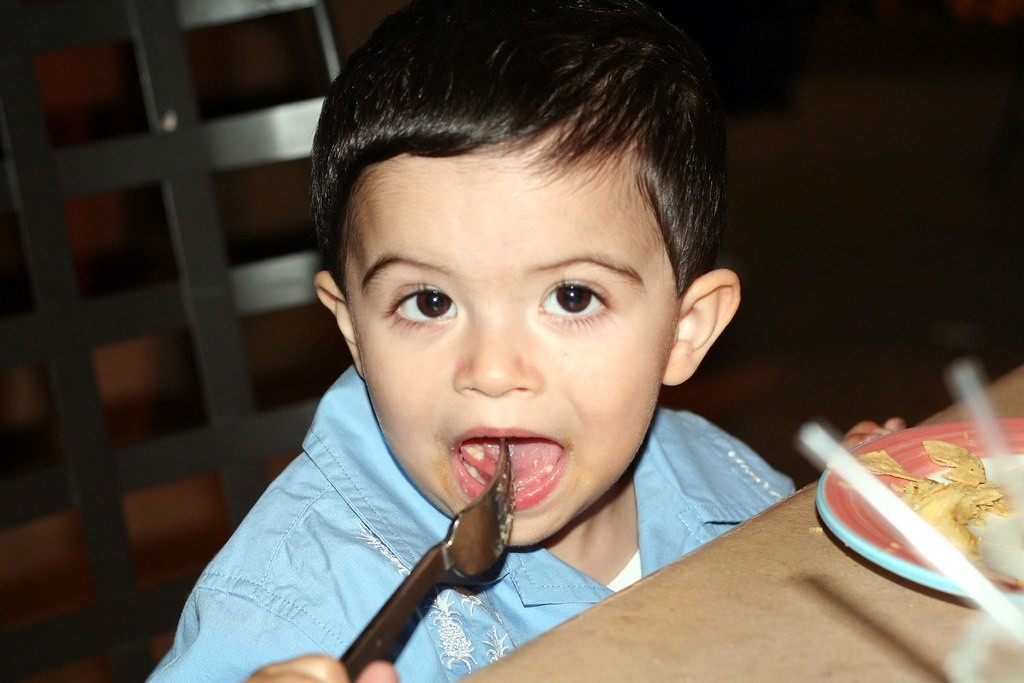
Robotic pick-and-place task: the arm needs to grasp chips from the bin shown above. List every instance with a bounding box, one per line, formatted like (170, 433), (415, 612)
(853, 440), (1018, 560)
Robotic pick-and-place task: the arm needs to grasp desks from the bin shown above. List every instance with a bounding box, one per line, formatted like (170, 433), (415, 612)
(451, 361), (1024, 683)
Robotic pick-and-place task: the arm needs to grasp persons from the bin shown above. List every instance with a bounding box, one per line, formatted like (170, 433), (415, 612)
(137, 0), (794, 683)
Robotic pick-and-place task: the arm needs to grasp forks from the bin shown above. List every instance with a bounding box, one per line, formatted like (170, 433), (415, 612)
(340, 438), (517, 683)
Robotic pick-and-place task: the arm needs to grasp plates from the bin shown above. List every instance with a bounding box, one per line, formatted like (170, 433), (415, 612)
(815, 416), (1024, 600)
